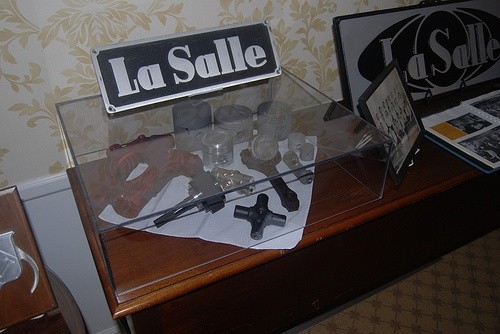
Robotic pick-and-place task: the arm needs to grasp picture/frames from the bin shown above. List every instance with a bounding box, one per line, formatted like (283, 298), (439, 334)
(358, 59), (425, 185)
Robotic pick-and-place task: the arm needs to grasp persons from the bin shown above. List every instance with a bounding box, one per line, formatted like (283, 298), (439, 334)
(375, 87), (417, 161)
